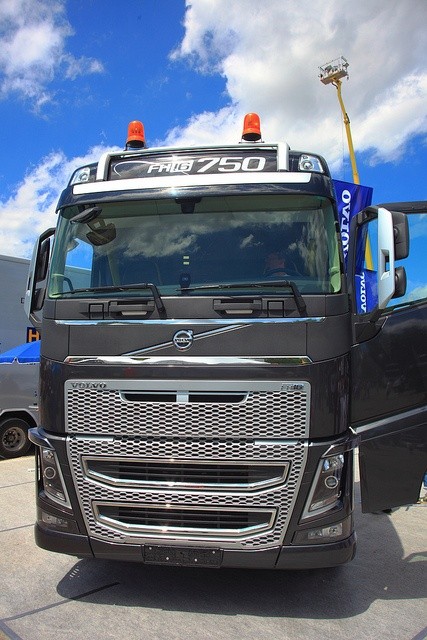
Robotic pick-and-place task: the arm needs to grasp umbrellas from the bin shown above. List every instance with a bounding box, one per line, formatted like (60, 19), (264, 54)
(0, 340), (41, 365)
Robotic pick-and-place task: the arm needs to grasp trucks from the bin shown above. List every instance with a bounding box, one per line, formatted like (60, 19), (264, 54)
(24, 113), (427, 570)
(0, 255), (92, 458)
